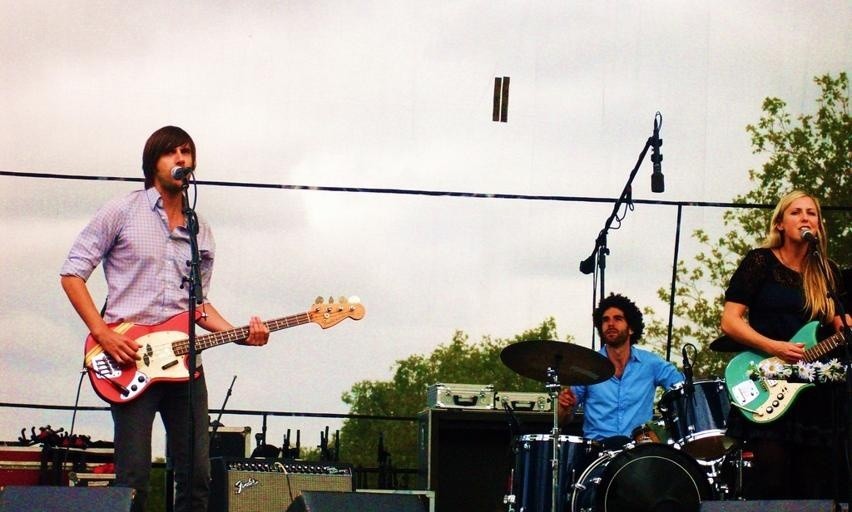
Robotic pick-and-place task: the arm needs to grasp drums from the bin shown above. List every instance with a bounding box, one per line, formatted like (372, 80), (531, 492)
(658, 378), (733, 450)
(503, 434), (606, 512)
(566, 440), (710, 512)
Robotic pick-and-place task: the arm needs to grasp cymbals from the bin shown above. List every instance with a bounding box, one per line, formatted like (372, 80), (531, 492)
(500, 340), (615, 386)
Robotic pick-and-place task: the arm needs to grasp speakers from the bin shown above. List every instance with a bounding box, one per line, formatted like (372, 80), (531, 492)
(286, 490), (426, 512)
(0, 485), (140, 512)
(700, 500), (838, 512)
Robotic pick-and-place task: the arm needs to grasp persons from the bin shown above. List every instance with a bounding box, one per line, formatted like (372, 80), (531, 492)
(59, 126), (270, 512)
(556, 292), (686, 442)
(719, 191), (852, 501)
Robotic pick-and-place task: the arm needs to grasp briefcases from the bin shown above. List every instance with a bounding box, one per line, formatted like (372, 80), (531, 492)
(495, 391), (552, 411)
(427, 383), (495, 410)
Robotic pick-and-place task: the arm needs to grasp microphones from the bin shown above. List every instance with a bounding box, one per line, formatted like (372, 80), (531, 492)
(171, 166), (195, 180)
(651, 119), (664, 192)
(682, 348), (694, 378)
(800, 229), (819, 245)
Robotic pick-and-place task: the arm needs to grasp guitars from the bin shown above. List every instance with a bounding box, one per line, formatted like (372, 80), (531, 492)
(84, 296), (365, 404)
(725, 320), (852, 425)
